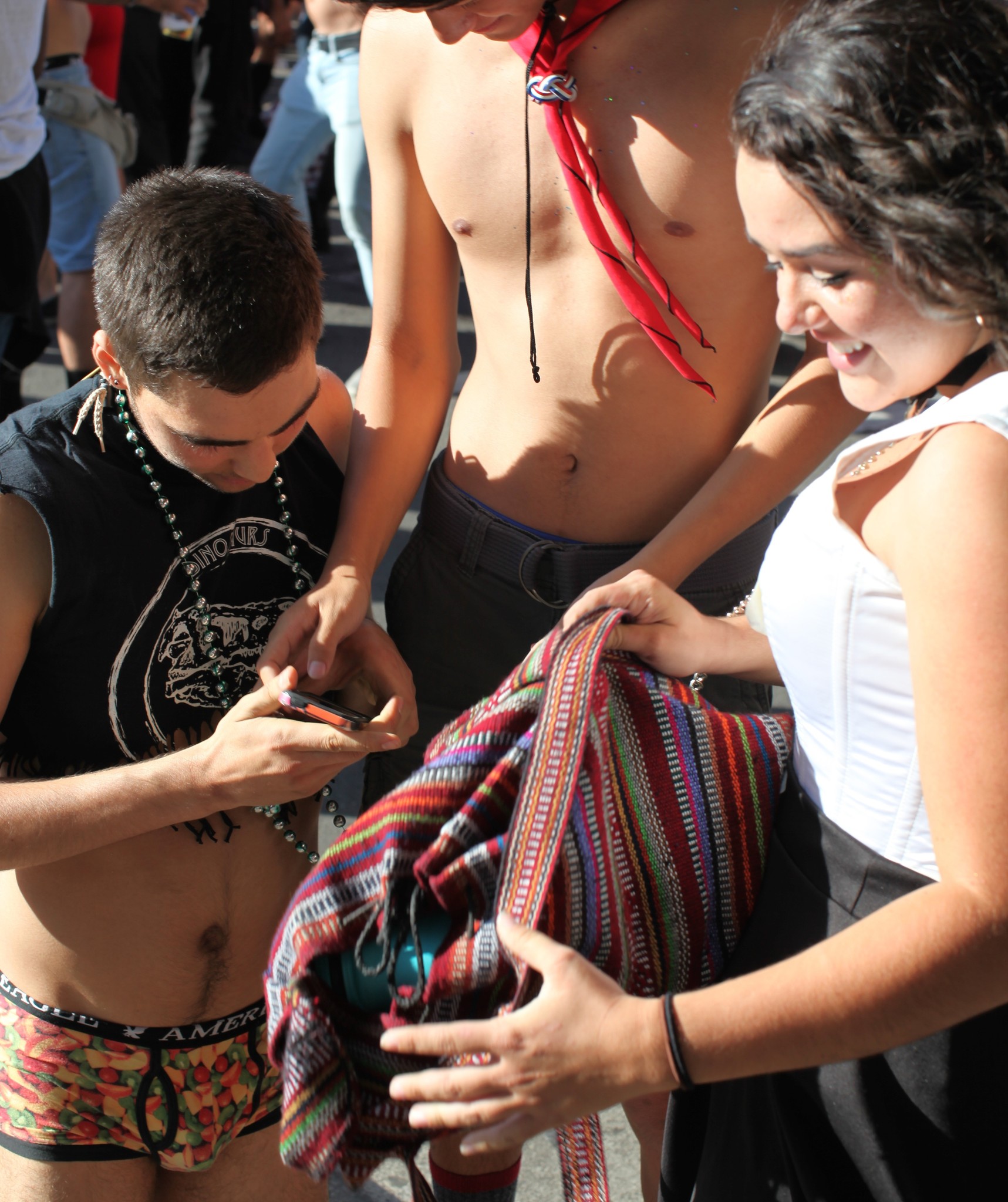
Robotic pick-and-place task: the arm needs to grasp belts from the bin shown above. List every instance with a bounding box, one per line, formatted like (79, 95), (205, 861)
(318, 30), (361, 52)
(40, 51), (79, 73)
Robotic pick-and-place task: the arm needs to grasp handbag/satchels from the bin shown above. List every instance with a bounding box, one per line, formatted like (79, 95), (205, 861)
(262, 603), (795, 1188)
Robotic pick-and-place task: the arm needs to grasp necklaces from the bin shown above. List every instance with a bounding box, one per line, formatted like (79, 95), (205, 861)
(115, 390), (344, 864)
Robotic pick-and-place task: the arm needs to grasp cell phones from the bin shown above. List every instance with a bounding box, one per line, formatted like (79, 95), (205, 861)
(278, 690), (372, 732)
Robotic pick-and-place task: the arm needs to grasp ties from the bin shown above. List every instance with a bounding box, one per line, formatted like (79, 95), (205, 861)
(507, 0), (719, 402)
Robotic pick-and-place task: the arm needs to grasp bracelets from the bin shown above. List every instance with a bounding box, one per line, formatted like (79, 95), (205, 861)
(660, 994), (680, 1084)
(666, 991), (688, 1084)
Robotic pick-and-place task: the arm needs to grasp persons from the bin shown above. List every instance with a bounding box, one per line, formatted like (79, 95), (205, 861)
(0, 0), (1008, 1202)
